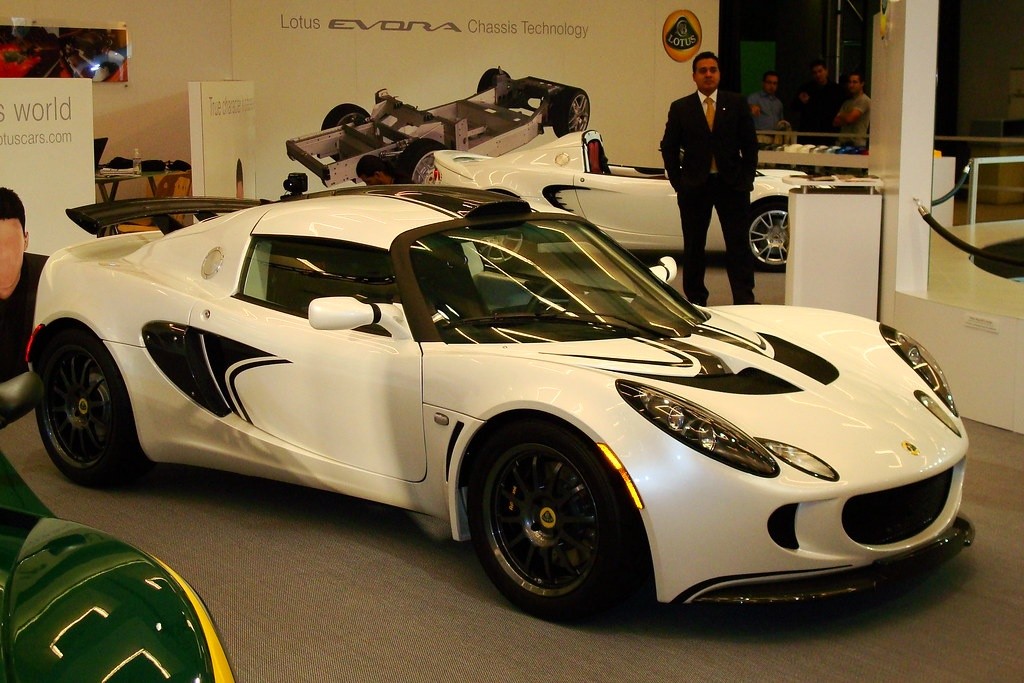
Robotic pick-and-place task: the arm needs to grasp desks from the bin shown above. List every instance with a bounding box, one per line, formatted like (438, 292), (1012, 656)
(759, 149), (870, 174)
(92, 164), (194, 238)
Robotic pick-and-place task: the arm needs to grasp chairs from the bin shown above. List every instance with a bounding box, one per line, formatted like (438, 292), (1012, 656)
(582, 129), (613, 176)
(410, 233), (495, 323)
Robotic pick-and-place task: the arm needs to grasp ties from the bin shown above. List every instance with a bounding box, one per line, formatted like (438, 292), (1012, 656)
(704, 97), (717, 173)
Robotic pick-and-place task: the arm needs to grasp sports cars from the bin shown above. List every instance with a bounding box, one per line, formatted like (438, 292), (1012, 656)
(1, 449), (237, 683)
(22, 183), (977, 623)
(409, 129), (816, 272)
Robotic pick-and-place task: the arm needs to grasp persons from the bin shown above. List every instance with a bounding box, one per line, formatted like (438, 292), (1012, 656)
(745, 58), (870, 147)
(355, 155), (418, 186)
(659, 50), (760, 306)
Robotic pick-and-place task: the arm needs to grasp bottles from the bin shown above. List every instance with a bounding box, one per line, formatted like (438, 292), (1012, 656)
(133, 148), (142, 176)
(165, 162), (175, 174)
